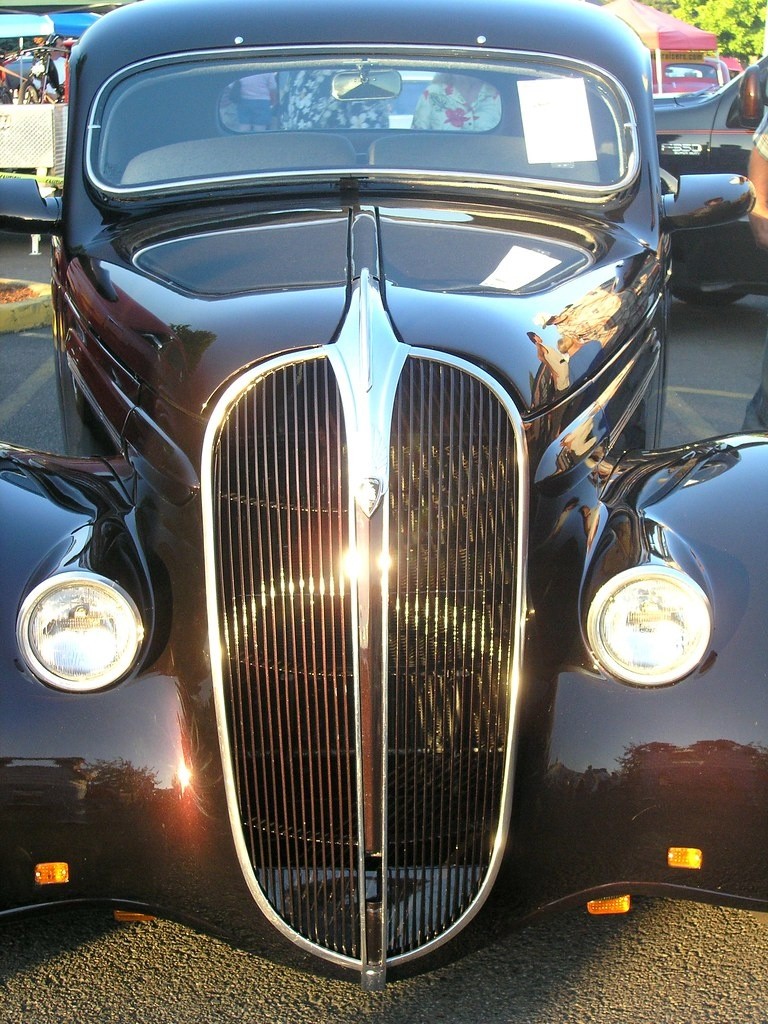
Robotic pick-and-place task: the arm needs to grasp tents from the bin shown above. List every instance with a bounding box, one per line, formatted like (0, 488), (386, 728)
(600, 0), (723, 96)
(0, 0), (106, 39)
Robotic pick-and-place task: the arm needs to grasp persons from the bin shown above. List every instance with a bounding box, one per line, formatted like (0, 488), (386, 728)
(32, 34), (68, 103)
(739, 112), (768, 437)
(410, 72), (502, 131)
(230, 66), (390, 132)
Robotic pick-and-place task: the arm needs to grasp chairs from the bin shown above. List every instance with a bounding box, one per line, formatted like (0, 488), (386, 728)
(367, 133), (599, 183)
(119, 130), (358, 185)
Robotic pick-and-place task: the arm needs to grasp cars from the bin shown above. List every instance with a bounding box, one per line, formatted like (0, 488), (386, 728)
(0, 1), (766, 1000)
